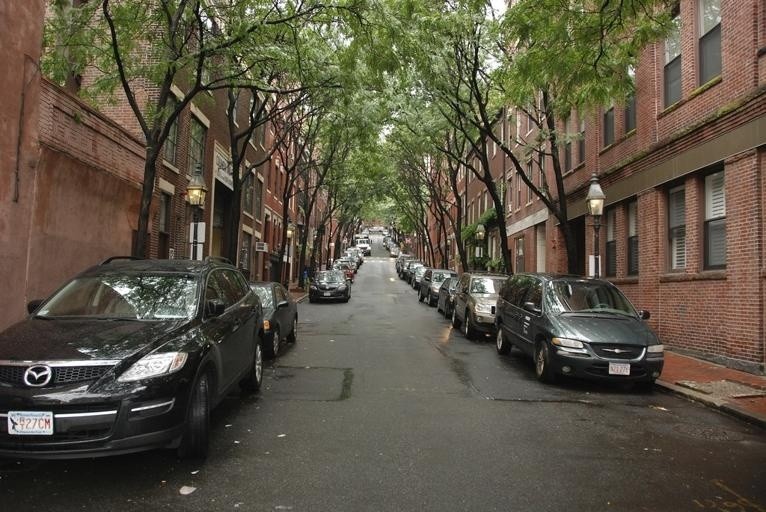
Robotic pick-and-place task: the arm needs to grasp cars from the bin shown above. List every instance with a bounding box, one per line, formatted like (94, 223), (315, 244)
(309, 238), (371, 303)
(248, 281), (297, 356)
(396, 256), (511, 337)
(382, 229), (400, 257)
(495, 272), (664, 384)
(0, 256), (264, 460)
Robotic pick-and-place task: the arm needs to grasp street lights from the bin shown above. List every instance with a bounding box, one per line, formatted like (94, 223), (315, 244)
(446, 232), (451, 270)
(585, 171), (606, 278)
(474, 223), (486, 271)
(186, 162), (209, 260)
(285, 217), (292, 290)
